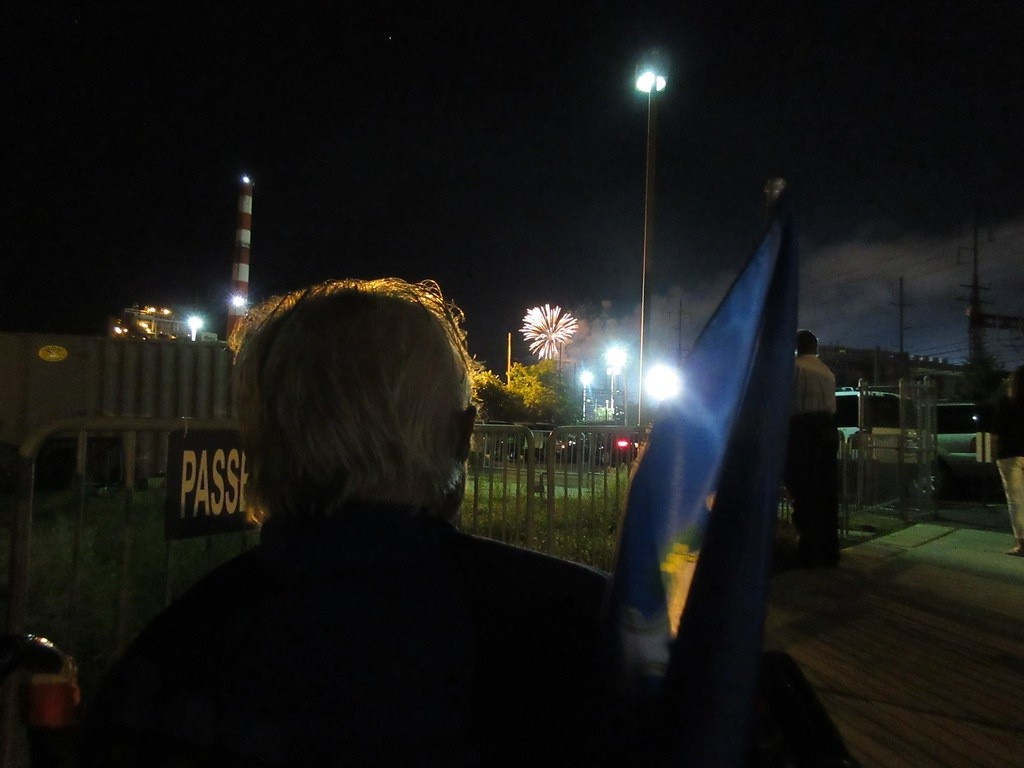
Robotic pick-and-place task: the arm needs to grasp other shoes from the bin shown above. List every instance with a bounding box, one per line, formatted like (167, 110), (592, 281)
(779, 551), (839, 569)
(1006, 545), (1024, 556)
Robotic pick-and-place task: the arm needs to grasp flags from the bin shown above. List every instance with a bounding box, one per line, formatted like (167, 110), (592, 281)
(596, 178), (798, 767)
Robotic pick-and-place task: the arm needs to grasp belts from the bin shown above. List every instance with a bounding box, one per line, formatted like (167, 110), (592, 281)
(793, 413), (834, 422)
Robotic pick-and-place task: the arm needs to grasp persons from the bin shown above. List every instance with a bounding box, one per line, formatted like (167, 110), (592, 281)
(83, 281), (615, 766)
(993, 365), (1024, 560)
(778, 327), (842, 566)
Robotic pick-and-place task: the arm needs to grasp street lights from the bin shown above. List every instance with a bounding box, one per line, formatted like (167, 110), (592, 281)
(581, 373), (593, 422)
(632, 71), (667, 425)
(608, 353), (625, 411)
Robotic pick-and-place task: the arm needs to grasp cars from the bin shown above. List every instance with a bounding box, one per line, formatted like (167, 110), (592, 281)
(917, 404), (987, 466)
(555, 420), (640, 466)
(832, 386), (922, 484)
